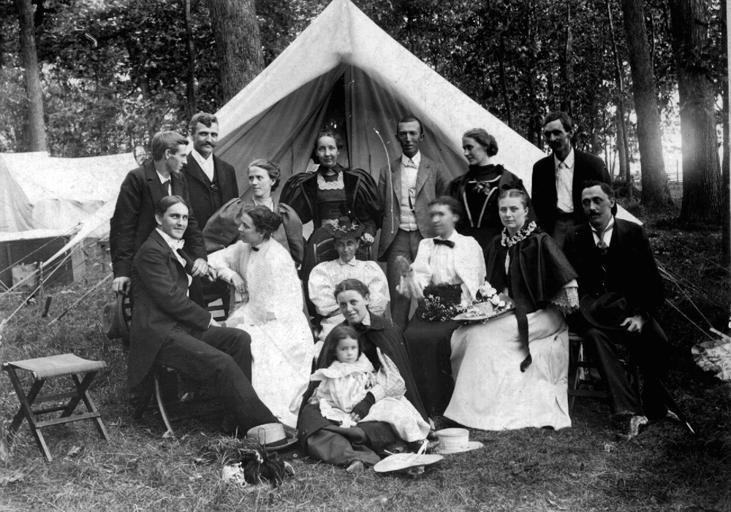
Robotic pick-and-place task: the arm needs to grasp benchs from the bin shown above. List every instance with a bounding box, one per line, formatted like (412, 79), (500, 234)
(117, 236), (612, 441)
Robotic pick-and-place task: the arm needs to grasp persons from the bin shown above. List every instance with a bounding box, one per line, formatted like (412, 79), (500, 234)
(556, 181), (666, 442)
(276, 126), (388, 331)
(307, 324), (429, 448)
(437, 188), (581, 433)
(199, 156), (305, 285)
(396, 197), (488, 414)
(124, 195), (292, 444)
(447, 128), (536, 262)
(174, 111), (239, 235)
(529, 112), (618, 384)
(295, 278), (430, 472)
(306, 224), (392, 360)
(205, 206), (316, 428)
(374, 116), (454, 333)
(109, 132), (210, 406)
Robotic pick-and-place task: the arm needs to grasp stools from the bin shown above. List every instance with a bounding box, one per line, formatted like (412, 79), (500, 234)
(1, 352), (109, 465)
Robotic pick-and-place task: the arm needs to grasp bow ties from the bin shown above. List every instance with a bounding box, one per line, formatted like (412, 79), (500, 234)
(433, 237), (455, 247)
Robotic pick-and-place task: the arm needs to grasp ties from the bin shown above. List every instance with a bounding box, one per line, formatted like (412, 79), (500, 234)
(163, 179), (171, 193)
(592, 225), (613, 249)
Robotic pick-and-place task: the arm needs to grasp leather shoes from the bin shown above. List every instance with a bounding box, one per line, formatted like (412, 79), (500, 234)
(346, 461), (364, 474)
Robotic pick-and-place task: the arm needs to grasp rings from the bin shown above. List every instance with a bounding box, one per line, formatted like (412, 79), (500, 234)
(239, 287), (243, 291)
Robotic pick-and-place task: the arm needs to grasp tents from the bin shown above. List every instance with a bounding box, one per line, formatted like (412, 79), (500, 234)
(177, 0), (647, 229)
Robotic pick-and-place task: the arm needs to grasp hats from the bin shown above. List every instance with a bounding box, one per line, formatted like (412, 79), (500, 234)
(432, 428), (483, 455)
(453, 292), (514, 321)
(240, 422), (298, 452)
(374, 451), (443, 474)
